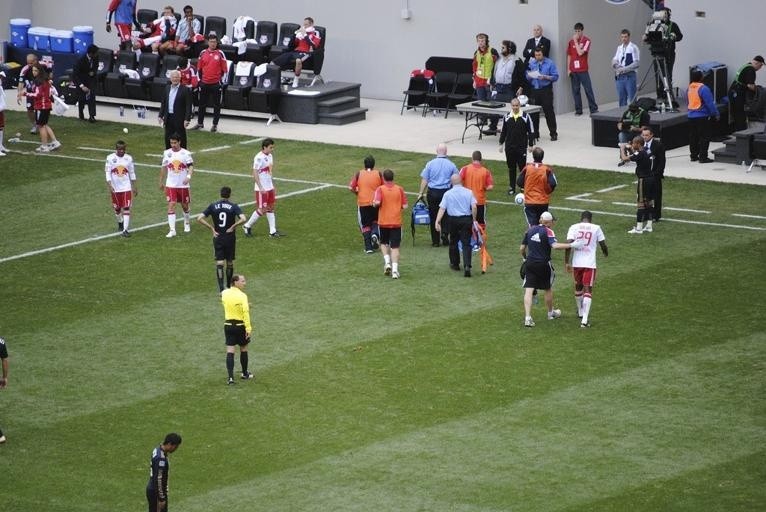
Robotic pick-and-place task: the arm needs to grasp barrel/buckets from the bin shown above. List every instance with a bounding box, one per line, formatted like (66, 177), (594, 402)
(72, 25), (94, 59)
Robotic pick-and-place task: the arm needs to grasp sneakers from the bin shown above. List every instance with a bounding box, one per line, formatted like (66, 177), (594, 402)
(441, 234), (449, 245)
(483, 129), (496, 134)
(371, 234), (379, 249)
(35, 145), (49, 152)
(551, 136), (557, 140)
(432, 243), (439, 247)
(548, 310), (561, 319)
(449, 264), (459, 270)
(524, 318), (536, 327)
(691, 157), (699, 161)
(166, 231), (176, 237)
(118, 221), (123, 231)
(227, 378), (234, 385)
(581, 322), (590, 327)
(269, 231), (286, 238)
(699, 159), (713, 163)
(31, 127), (37, 134)
(292, 75), (300, 88)
(463, 266), (471, 276)
(366, 250), (372, 253)
(392, 271), (400, 279)
(242, 225), (252, 237)
(627, 227), (644, 234)
(240, 371), (254, 380)
(122, 231), (131, 237)
(47, 141), (61, 151)
(184, 225), (190, 232)
(383, 263), (391, 275)
(643, 227), (653, 232)
(88, 117), (96, 122)
(211, 125), (217, 131)
(189, 125), (203, 129)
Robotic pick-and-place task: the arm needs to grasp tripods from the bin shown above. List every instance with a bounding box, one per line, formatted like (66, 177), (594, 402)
(629, 57), (679, 113)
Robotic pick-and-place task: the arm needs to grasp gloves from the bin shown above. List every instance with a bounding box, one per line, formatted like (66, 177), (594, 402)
(571, 239), (585, 249)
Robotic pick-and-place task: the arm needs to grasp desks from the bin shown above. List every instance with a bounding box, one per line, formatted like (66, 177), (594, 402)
(454, 100), (543, 147)
(4, 44), (80, 79)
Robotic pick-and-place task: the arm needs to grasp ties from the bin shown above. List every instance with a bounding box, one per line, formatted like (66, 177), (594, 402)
(646, 144), (648, 147)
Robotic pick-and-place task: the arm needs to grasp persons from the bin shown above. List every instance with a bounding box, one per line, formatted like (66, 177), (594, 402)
(241, 139), (285, 237)
(499, 98), (533, 195)
(418, 144), (495, 277)
(566, 23), (598, 115)
(220, 274), (254, 385)
(616, 104), (666, 235)
(350, 154), (408, 279)
(686, 71), (720, 163)
(1, 0), (321, 154)
(728, 55), (766, 131)
(517, 147), (558, 229)
(473, 23), (560, 143)
(565, 211), (609, 329)
(105, 140), (138, 237)
(519, 212), (584, 327)
(146, 429), (181, 511)
(160, 133), (194, 238)
(642, 7), (682, 104)
(612, 29), (641, 106)
(198, 186), (246, 290)
(0, 334), (11, 444)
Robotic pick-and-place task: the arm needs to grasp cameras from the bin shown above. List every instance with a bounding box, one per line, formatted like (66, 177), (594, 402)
(617, 142), (632, 150)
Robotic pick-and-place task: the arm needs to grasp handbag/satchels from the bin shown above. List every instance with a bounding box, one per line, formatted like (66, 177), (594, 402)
(411, 196), (431, 225)
(458, 223), (482, 252)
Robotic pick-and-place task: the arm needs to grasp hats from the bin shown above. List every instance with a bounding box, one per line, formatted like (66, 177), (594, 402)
(753, 56), (766, 65)
(541, 212), (559, 221)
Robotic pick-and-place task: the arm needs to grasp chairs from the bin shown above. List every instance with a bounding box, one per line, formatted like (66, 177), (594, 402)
(88, 7), (327, 129)
(471, 89), (480, 102)
(425, 71), (463, 120)
(446, 73), (476, 122)
(400, 70), (436, 117)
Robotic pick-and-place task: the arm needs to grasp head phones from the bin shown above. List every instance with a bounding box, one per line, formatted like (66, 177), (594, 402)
(508, 41), (513, 53)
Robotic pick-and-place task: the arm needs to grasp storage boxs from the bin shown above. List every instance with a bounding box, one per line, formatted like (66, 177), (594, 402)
(73, 25), (95, 57)
(49, 30), (76, 53)
(28, 26), (57, 52)
